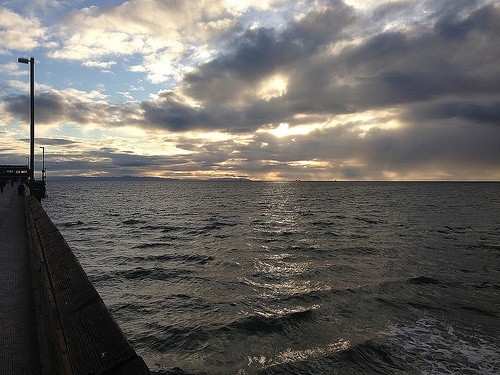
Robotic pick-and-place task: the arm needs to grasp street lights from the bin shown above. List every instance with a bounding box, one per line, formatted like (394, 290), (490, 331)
(40, 147), (45, 181)
(16, 56), (35, 181)
